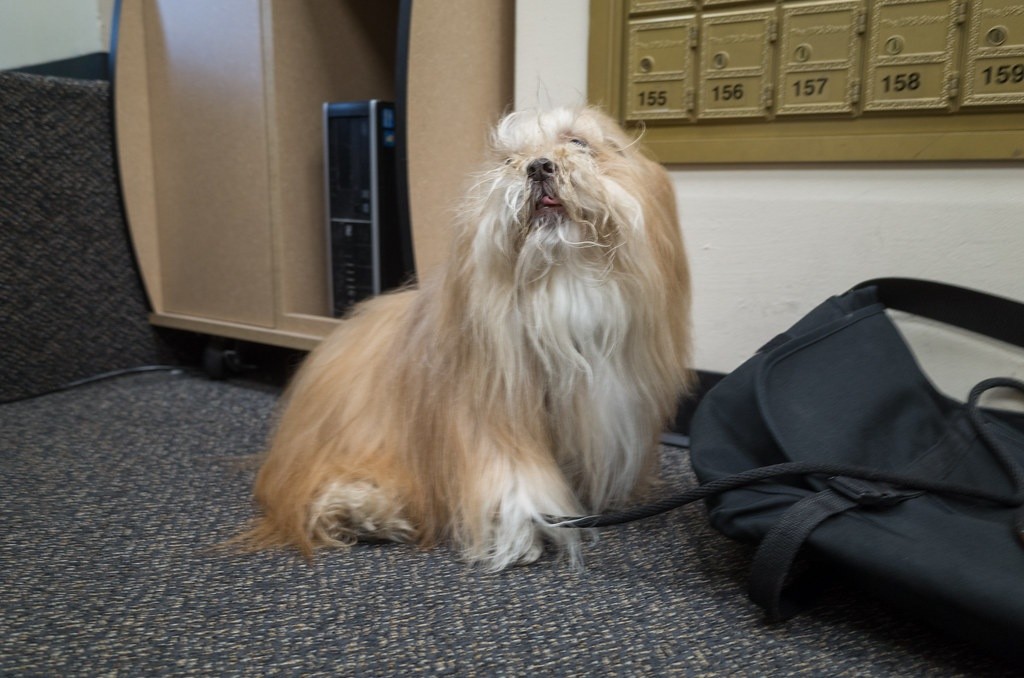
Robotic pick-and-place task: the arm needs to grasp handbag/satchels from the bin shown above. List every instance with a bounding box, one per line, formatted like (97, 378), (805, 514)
(688, 274), (1024, 678)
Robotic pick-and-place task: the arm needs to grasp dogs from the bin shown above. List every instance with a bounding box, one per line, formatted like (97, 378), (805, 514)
(211, 100), (694, 568)
